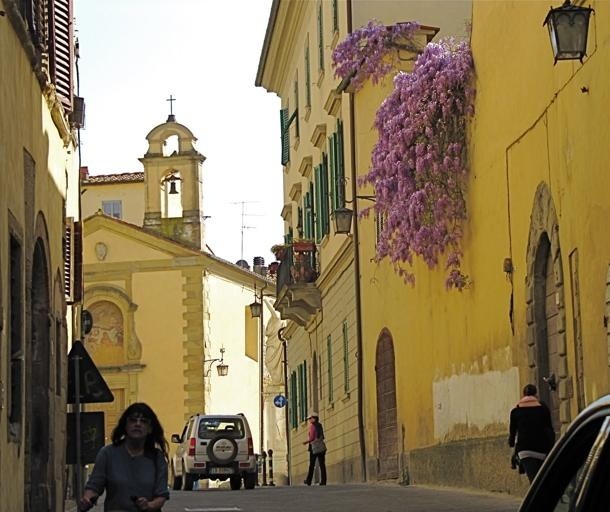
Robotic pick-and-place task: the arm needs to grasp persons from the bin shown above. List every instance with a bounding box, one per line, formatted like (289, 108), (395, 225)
(302, 412), (327, 486)
(508, 384), (555, 484)
(80, 403), (170, 512)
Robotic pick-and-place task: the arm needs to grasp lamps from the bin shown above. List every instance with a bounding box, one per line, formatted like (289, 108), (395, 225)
(543, 0), (594, 65)
(329, 177), (376, 237)
(204, 345), (228, 377)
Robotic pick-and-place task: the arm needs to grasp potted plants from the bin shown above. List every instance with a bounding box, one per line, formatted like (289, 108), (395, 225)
(271, 243), (288, 260)
(293, 237), (315, 252)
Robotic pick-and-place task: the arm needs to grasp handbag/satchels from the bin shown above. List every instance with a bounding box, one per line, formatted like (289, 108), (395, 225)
(310, 435), (327, 454)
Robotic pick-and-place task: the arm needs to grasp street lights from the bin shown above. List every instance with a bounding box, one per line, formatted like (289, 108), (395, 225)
(166, 412), (258, 490)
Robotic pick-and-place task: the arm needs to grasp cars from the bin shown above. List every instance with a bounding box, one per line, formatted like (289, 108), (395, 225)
(510, 396), (609, 510)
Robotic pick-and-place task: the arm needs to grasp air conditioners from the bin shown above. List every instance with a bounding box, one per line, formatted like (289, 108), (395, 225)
(69, 97), (85, 128)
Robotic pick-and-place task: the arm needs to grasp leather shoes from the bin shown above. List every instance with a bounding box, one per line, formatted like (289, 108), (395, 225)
(304, 479), (311, 485)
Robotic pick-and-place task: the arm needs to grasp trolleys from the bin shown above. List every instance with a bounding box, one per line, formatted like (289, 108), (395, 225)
(81, 491), (149, 512)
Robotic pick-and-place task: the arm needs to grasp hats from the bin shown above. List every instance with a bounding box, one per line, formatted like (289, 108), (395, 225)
(307, 412), (318, 419)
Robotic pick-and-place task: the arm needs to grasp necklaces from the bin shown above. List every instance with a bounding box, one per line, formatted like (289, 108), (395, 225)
(126, 445), (144, 459)
